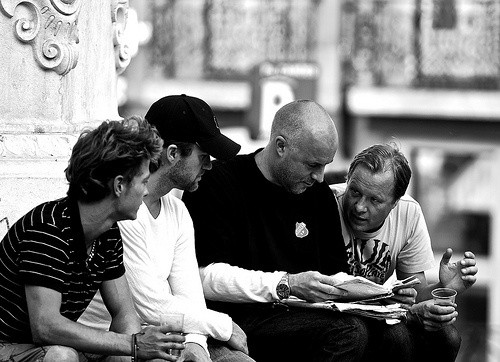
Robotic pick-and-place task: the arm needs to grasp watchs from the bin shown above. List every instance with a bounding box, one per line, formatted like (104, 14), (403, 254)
(277, 273), (290, 299)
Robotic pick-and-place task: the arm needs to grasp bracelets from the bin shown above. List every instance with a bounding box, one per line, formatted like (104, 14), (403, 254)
(131, 332), (146, 361)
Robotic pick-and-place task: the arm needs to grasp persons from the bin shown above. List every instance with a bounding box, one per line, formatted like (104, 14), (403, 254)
(1, 116), (186, 362)
(76, 95), (256, 362)
(181, 99), (418, 362)
(330, 144), (477, 362)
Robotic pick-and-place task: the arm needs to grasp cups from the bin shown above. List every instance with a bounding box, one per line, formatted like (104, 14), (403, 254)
(158, 312), (185, 361)
(428, 286), (458, 328)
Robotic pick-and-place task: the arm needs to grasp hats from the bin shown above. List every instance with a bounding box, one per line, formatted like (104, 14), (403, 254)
(144, 93), (242, 159)
